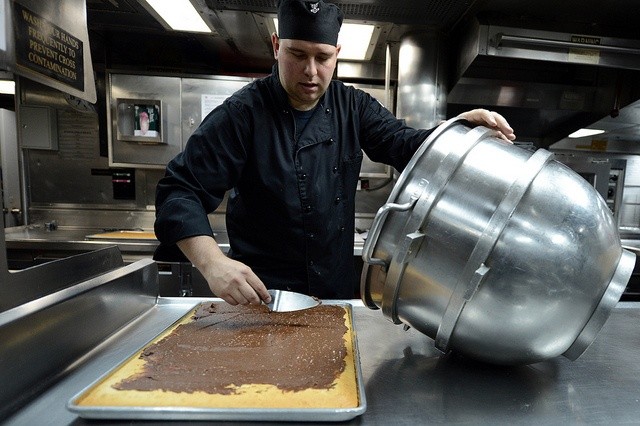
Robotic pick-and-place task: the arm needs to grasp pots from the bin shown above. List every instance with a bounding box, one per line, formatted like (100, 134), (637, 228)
(360, 117), (637, 365)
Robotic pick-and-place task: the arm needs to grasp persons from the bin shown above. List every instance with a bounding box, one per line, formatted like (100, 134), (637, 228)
(134, 111), (158, 137)
(154, 1), (517, 306)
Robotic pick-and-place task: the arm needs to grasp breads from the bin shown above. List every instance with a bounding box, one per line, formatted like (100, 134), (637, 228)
(75, 299), (360, 411)
(97, 229), (159, 241)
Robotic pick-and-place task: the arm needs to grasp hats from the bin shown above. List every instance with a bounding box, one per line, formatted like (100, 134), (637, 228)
(278, 0), (343, 46)
